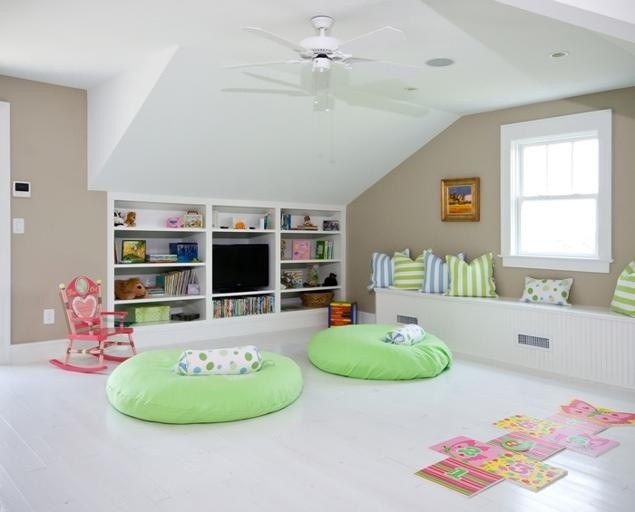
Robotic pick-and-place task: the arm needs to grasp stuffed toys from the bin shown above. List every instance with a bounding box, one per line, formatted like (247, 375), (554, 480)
(115, 278), (146, 300)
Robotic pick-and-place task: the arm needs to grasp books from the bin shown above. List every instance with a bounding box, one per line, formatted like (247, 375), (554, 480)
(292, 239), (311, 260)
(142, 269), (201, 299)
(145, 253), (178, 263)
(212, 294), (274, 318)
(297, 225), (319, 230)
(315, 239), (333, 259)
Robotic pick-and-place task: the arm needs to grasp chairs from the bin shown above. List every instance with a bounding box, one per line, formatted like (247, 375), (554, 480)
(49, 276), (136, 374)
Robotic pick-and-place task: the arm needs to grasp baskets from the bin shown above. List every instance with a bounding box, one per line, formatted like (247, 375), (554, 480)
(301, 291), (334, 309)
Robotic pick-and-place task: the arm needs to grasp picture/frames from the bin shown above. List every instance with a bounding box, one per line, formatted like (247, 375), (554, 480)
(440, 177), (480, 222)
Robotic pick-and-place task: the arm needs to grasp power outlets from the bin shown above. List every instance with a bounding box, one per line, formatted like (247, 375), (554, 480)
(43, 309), (54, 324)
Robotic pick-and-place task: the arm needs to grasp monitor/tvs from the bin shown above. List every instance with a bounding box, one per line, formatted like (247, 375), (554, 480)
(212, 243), (270, 293)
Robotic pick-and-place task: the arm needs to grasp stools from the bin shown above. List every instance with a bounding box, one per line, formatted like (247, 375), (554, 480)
(307, 323), (452, 380)
(107, 347), (305, 424)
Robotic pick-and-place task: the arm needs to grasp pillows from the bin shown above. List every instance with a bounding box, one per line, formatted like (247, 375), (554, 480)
(366, 249), (409, 291)
(518, 276), (574, 307)
(384, 324), (426, 346)
(420, 250), (465, 293)
(389, 252), (425, 290)
(178, 346), (261, 375)
(609, 262), (633, 319)
(443, 252), (498, 297)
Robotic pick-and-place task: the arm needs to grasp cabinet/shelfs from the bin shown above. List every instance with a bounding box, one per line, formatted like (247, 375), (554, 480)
(279, 208), (343, 310)
(112, 199), (207, 322)
(209, 204), (276, 319)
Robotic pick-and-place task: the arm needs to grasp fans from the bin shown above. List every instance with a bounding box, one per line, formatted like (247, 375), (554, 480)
(222, 24), (401, 74)
